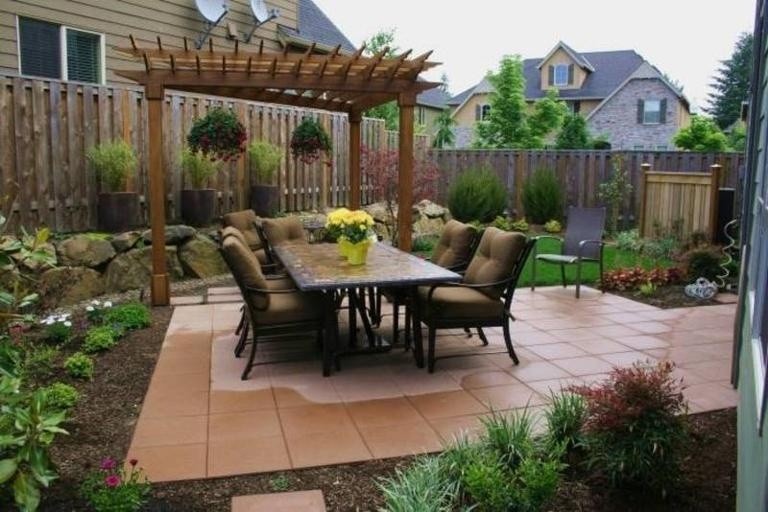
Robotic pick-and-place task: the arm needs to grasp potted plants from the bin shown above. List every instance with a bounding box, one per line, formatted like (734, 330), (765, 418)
(327, 208), (351, 257)
(85, 140), (143, 232)
(179, 152), (220, 226)
(248, 143), (284, 216)
(345, 209), (375, 265)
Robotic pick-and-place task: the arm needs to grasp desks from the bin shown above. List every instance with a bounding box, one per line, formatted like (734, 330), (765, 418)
(270, 230), (465, 378)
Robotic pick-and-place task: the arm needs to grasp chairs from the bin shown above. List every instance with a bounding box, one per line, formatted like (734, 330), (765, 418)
(371, 218), (491, 349)
(220, 214), (343, 381)
(532, 207), (606, 298)
(407, 225), (531, 376)
